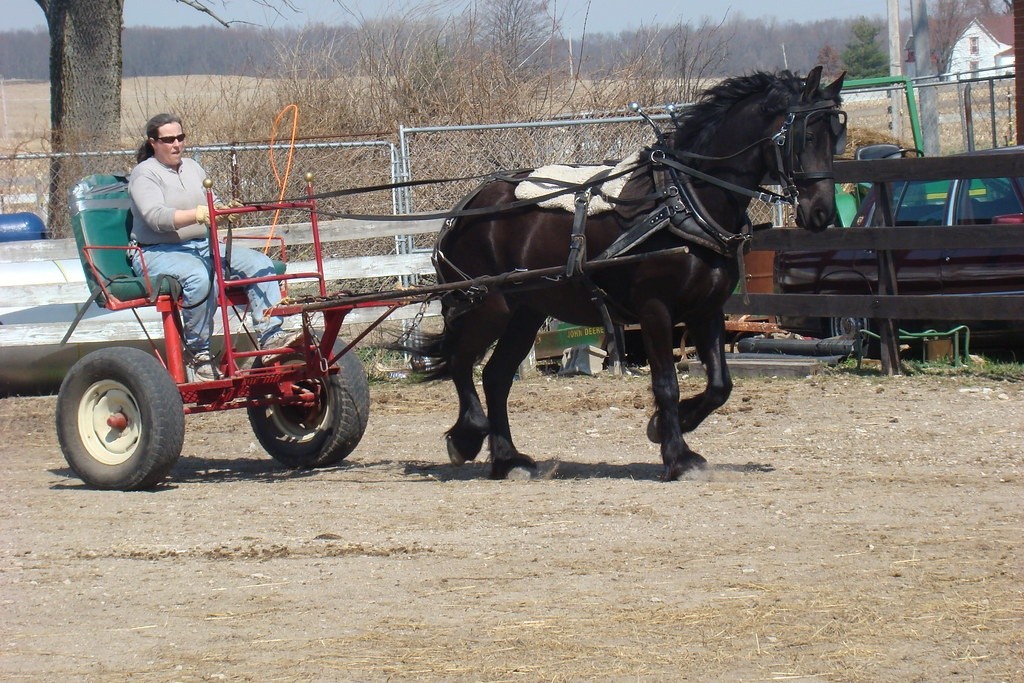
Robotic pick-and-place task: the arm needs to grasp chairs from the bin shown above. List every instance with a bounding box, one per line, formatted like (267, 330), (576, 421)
(69, 173), (286, 312)
(857, 145), (926, 208)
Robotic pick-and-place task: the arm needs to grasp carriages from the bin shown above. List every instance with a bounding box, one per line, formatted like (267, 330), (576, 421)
(54, 61), (848, 491)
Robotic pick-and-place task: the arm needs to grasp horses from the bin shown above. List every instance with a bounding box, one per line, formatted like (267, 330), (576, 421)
(428, 63), (850, 485)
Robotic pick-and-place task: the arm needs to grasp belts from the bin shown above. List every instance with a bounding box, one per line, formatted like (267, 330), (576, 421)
(137, 237), (207, 247)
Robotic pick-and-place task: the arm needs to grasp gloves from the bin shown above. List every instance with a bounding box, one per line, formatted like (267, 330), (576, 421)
(196, 204), (229, 226)
(226, 199), (245, 222)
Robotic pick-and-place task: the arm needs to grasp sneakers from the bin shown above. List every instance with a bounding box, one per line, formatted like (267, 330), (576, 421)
(260, 329), (304, 366)
(183, 347), (224, 381)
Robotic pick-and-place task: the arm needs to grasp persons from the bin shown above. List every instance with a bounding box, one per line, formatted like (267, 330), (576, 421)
(126, 114), (313, 383)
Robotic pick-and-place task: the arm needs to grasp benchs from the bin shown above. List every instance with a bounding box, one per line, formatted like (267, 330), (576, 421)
(892, 199), (989, 224)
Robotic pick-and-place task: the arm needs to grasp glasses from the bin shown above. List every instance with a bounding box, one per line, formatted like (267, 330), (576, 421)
(156, 133), (185, 143)
